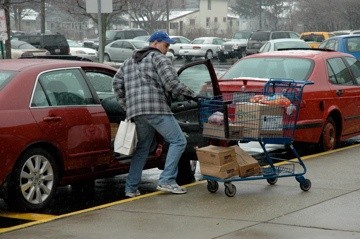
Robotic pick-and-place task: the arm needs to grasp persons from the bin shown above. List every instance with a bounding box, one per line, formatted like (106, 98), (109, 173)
(111, 29), (211, 199)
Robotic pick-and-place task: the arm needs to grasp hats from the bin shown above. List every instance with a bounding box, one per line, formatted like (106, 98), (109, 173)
(148, 31), (177, 44)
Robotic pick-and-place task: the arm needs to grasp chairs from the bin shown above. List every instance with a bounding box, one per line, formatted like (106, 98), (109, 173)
(46, 80), (84, 104)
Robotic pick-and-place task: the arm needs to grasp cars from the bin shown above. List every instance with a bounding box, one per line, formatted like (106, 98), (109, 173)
(68, 40), (100, 60)
(178, 37), (226, 59)
(200, 50), (360, 154)
(168, 35), (191, 57)
(258, 39), (312, 53)
(317, 36), (360, 61)
(0, 39), (50, 60)
(104, 40), (174, 62)
(0, 59), (221, 211)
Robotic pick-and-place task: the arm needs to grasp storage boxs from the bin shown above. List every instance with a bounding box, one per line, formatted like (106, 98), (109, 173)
(196, 102), (284, 179)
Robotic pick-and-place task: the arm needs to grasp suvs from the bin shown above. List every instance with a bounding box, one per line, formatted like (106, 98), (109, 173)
(83, 28), (150, 50)
(245, 31), (301, 56)
(21, 33), (70, 59)
(216, 32), (252, 62)
(300, 30), (360, 48)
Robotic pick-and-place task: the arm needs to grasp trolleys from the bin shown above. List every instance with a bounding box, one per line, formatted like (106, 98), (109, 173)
(197, 79), (316, 197)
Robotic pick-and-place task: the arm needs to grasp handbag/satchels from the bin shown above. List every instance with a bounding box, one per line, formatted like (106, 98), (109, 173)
(113, 118), (139, 156)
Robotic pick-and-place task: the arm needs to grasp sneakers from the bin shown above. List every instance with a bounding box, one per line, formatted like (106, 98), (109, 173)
(125, 189), (140, 198)
(157, 181), (188, 194)
